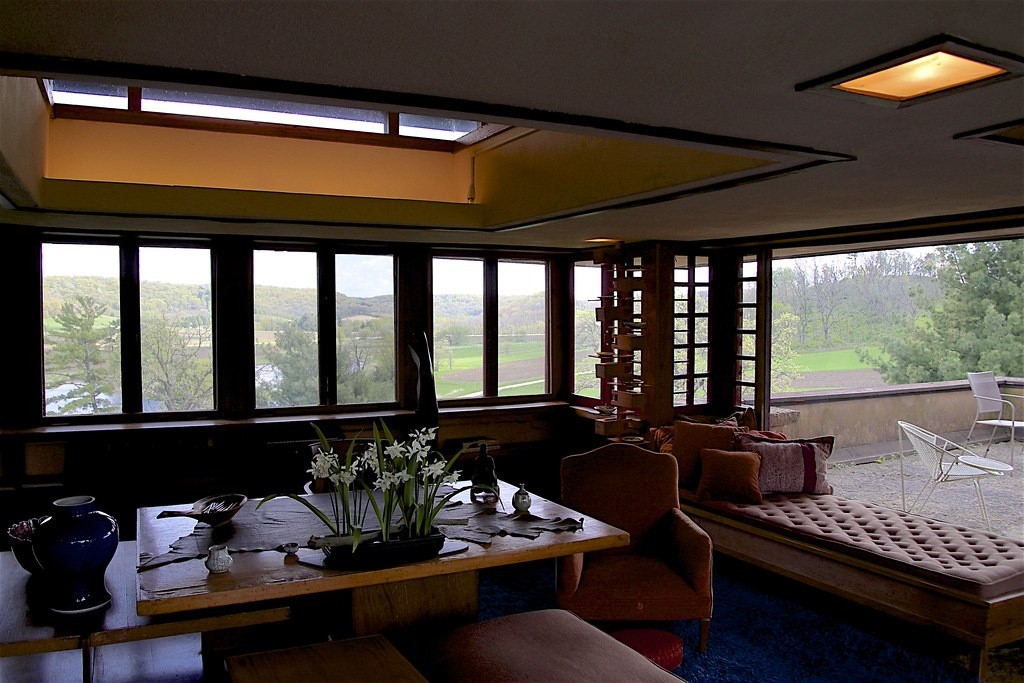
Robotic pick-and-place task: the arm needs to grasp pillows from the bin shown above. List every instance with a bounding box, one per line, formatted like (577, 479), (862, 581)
(648, 408), (835, 503)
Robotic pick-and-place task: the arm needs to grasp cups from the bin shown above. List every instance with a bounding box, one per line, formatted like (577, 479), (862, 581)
(283, 542), (300, 554)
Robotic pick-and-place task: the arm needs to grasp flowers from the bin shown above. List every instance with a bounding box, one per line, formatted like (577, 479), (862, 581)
(256, 418), (507, 553)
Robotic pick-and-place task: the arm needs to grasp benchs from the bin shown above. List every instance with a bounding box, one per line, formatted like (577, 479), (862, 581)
(678, 488), (1024, 683)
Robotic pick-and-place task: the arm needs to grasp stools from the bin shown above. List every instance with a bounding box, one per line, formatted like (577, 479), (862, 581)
(223, 633), (428, 683)
(443, 609), (690, 683)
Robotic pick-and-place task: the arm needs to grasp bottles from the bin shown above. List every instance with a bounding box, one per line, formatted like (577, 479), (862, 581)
(31, 495), (119, 614)
(512, 483), (531, 511)
(470, 443), (498, 502)
(205, 544), (232, 573)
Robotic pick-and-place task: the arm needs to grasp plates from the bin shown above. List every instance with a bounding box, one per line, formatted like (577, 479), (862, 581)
(594, 406), (617, 414)
(620, 436), (644, 442)
(596, 352), (615, 355)
(597, 296), (615, 298)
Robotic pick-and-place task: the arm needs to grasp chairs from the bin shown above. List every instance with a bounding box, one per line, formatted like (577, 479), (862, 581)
(898, 420), (1014, 532)
(560, 442), (715, 652)
(967, 371), (1024, 476)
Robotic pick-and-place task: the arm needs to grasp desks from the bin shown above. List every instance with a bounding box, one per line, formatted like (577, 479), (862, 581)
(136, 478), (630, 639)
(0, 540), (292, 683)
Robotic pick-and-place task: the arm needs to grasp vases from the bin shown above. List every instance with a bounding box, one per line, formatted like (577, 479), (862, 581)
(31, 497), (121, 614)
(322, 523), (446, 572)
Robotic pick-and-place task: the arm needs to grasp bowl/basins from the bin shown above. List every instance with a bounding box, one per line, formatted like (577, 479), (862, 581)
(623, 322), (646, 328)
(7, 516), (50, 575)
